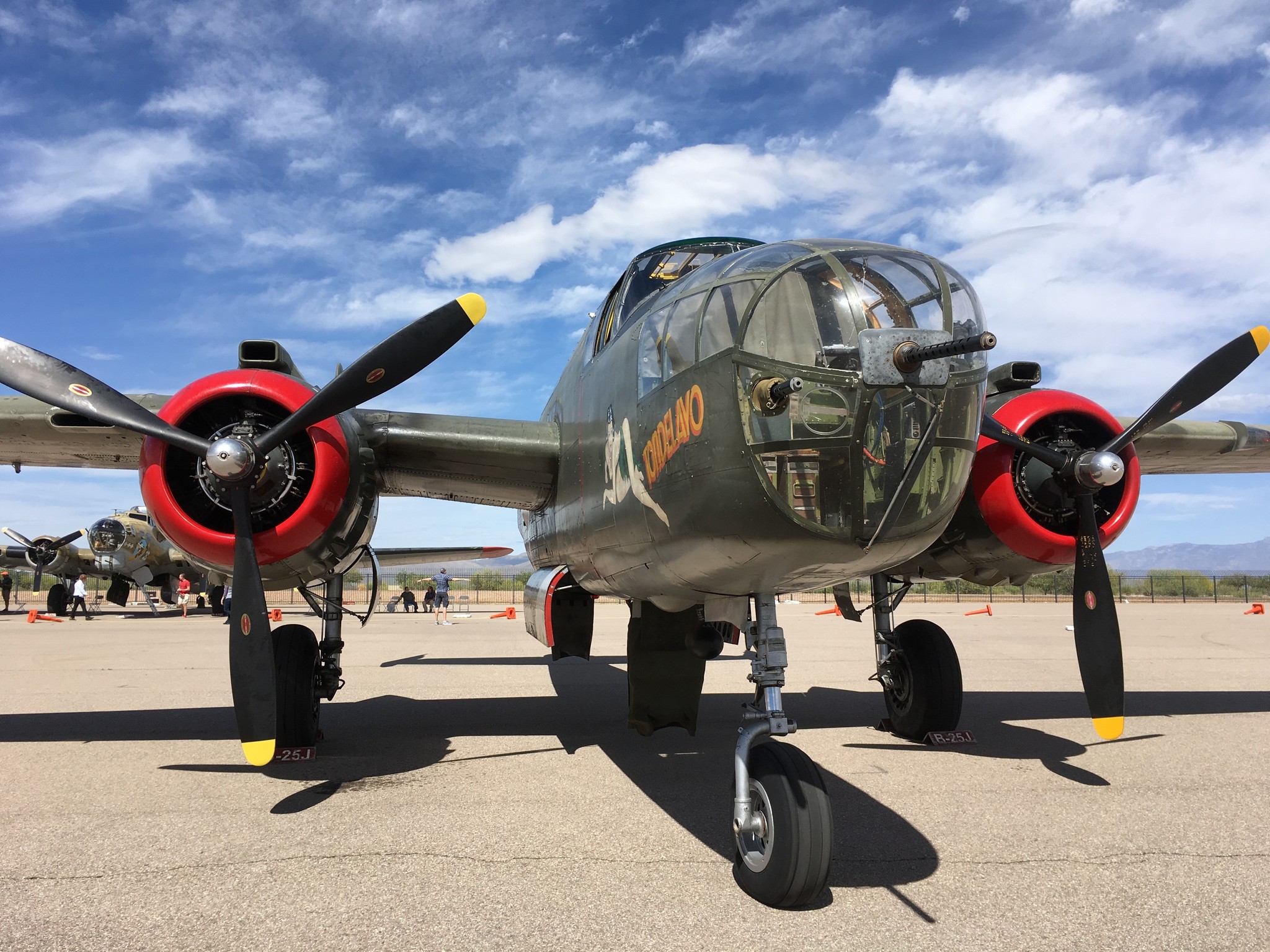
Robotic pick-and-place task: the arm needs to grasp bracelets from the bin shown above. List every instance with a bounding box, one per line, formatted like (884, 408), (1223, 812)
(421, 579), (422, 581)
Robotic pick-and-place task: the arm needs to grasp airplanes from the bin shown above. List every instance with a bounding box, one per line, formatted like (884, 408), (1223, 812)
(0, 237), (1270, 911)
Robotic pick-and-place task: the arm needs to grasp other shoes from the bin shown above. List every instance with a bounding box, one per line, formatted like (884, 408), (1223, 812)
(223, 621), (230, 625)
(429, 610), (432, 612)
(423, 611), (427, 613)
(414, 611), (419, 613)
(85, 616), (94, 620)
(0, 609), (8, 613)
(407, 611), (409, 613)
(182, 614), (187, 618)
(69, 618), (76, 621)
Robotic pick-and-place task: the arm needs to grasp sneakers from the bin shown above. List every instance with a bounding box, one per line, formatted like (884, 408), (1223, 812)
(442, 621), (453, 625)
(434, 621), (439, 625)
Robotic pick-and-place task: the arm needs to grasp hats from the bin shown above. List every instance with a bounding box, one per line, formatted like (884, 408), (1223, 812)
(440, 568), (446, 573)
(0, 571), (9, 575)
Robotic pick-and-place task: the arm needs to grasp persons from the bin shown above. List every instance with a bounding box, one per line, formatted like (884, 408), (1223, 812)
(417, 568), (470, 625)
(221, 585), (232, 624)
(422, 586), (435, 613)
(395, 587), (419, 613)
(0, 571), (13, 611)
(69, 574), (94, 620)
(176, 573), (190, 618)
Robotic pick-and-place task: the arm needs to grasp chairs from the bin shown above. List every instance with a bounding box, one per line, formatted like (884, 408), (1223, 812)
(87, 595), (104, 614)
(375, 596), (414, 613)
(65, 602), (77, 613)
(13, 595), (27, 614)
(426, 596), (469, 612)
(146, 591), (159, 603)
